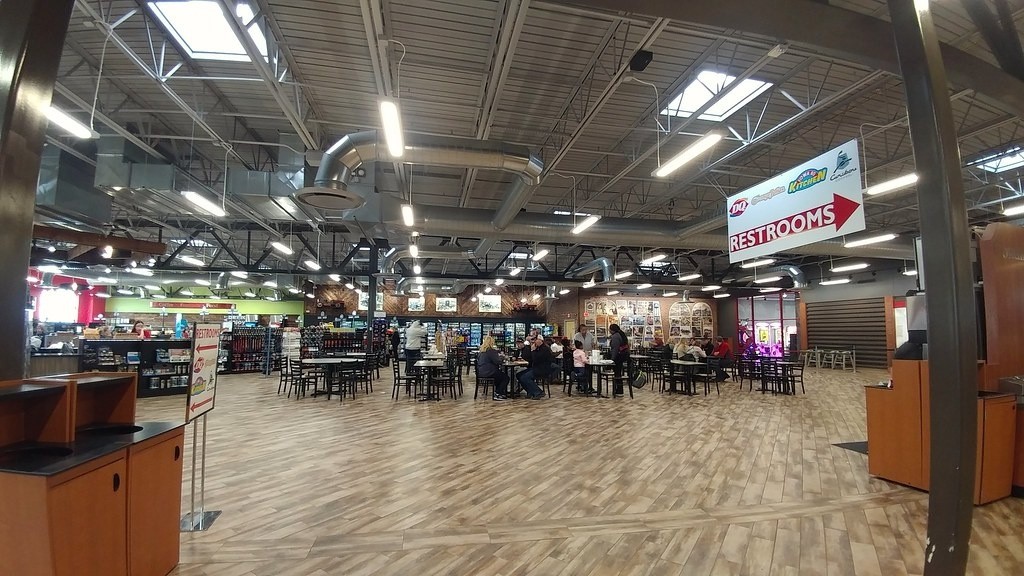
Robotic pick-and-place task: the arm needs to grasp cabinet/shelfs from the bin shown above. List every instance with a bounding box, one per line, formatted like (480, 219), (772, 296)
(78, 338), (192, 398)
(864, 360), (1017, 506)
(0, 372), (189, 576)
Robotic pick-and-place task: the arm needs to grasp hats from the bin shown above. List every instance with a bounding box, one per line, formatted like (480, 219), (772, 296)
(514, 338), (524, 344)
(531, 334), (544, 341)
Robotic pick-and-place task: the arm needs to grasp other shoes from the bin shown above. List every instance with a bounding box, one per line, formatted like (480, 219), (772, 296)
(532, 391), (545, 400)
(579, 389), (591, 393)
(493, 391), (508, 401)
(722, 375), (730, 380)
(525, 393), (532, 399)
(615, 393), (624, 397)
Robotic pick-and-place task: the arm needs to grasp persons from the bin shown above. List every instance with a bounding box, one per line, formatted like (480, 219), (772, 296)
(665, 336), (674, 349)
(131, 321), (150, 339)
(280, 319), (288, 328)
(574, 324), (594, 391)
(435, 324), (469, 354)
(573, 340), (590, 394)
(389, 326), (400, 361)
(673, 336), (734, 383)
(653, 334), (663, 348)
(476, 329), (563, 400)
(161, 331), (165, 337)
(404, 320), (428, 391)
(183, 325), (191, 339)
(609, 324), (628, 397)
(561, 338), (575, 373)
(33, 320), (46, 348)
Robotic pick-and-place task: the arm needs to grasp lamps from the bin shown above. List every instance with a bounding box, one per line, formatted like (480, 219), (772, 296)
(643, 253), (669, 265)
(739, 258), (777, 269)
(650, 129), (730, 178)
(46, 95), (415, 226)
(677, 273), (702, 281)
(613, 269), (634, 279)
(753, 260), (783, 283)
(818, 263), (852, 285)
(828, 255), (871, 272)
(713, 291), (731, 298)
(841, 227), (901, 248)
(902, 260), (916, 276)
(867, 173), (918, 196)
(702, 278), (722, 292)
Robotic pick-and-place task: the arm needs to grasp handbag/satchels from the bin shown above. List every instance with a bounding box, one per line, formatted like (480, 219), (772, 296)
(632, 370), (646, 388)
(613, 335), (629, 354)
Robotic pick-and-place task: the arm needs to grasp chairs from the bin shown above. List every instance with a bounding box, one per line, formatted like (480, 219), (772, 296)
(277, 347), (806, 402)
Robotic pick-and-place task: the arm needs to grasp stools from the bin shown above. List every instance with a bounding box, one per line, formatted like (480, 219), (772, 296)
(808, 350), (824, 367)
(820, 351), (840, 370)
(832, 351), (855, 371)
(798, 349), (813, 365)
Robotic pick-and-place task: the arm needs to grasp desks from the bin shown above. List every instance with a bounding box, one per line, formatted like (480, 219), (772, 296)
(766, 361), (805, 395)
(629, 354), (651, 371)
(676, 361), (705, 396)
(422, 355), (445, 378)
(584, 362), (615, 399)
(416, 360), (443, 397)
(414, 363), (443, 402)
(664, 360), (686, 393)
(501, 360), (528, 400)
(295, 358), (365, 400)
